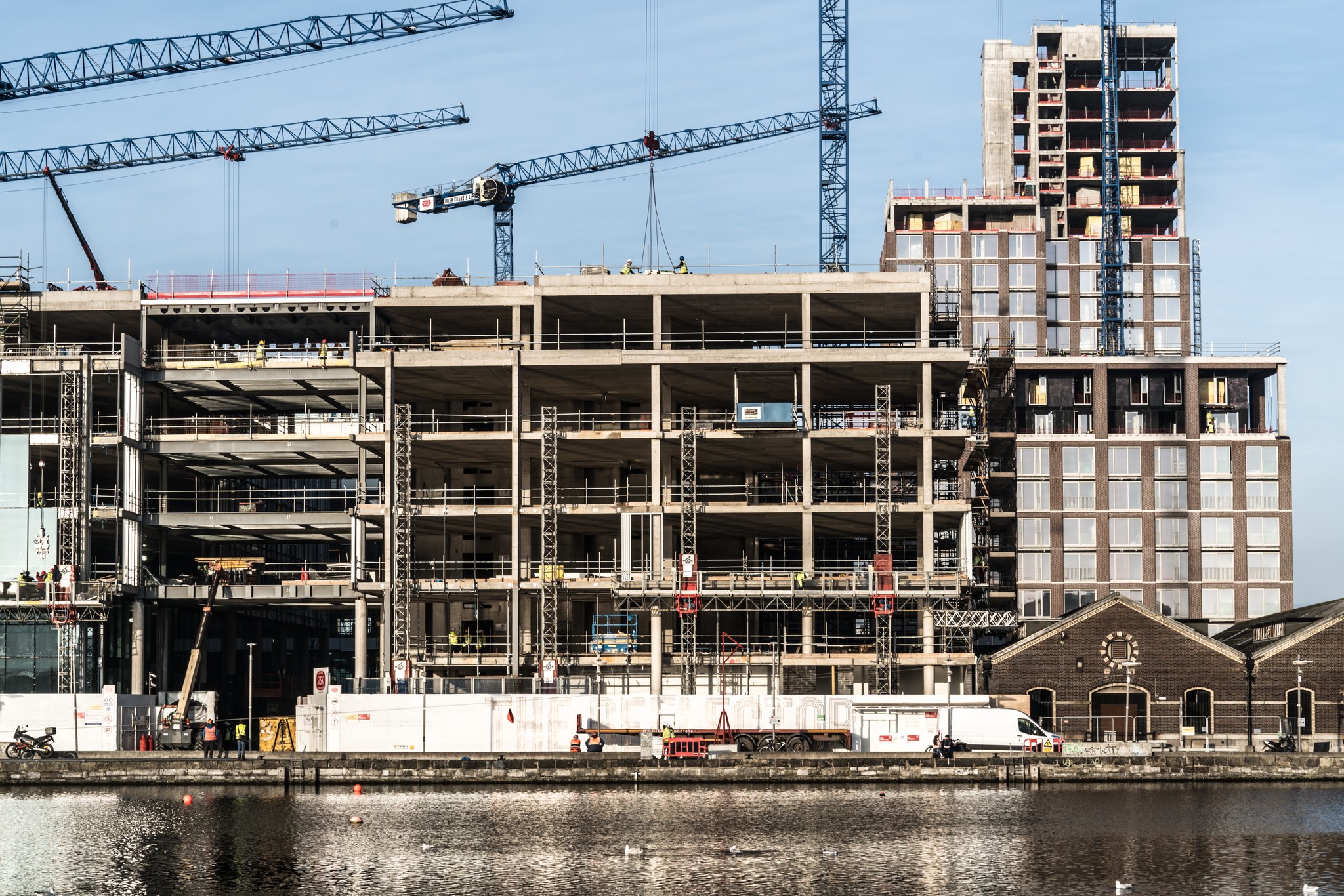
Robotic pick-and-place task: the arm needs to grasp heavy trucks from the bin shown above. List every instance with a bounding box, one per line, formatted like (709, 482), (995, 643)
(243, 673), (311, 716)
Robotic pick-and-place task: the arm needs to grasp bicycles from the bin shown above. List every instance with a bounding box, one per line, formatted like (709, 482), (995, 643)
(756, 738), (786, 752)
(759, 737), (795, 752)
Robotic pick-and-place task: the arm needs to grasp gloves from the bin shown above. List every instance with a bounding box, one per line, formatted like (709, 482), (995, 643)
(36, 572), (40, 575)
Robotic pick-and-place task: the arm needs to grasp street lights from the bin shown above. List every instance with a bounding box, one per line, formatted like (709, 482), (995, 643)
(1243, 648), (1255, 745)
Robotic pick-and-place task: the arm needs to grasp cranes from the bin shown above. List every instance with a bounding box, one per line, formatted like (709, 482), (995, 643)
(150, 566), (232, 751)
(0, 0), (516, 299)
(385, 0), (880, 289)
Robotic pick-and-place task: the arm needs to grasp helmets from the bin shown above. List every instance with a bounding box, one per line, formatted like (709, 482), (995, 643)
(259, 341), (264, 344)
(949, 560), (953, 563)
(627, 259), (632, 262)
(973, 549), (978, 553)
(337, 344), (342, 347)
(450, 627), (484, 635)
(663, 722), (670, 727)
(208, 719), (231, 727)
(680, 256), (684, 259)
(322, 339), (327, 343)
(41, 568), (55, 575)
(19, 570), (25, 573)
(973, 407), (975, 410)
(805, 572), (810, 578)
(572, 731), (597, 738)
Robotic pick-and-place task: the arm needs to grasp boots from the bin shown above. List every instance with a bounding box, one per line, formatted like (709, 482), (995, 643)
(238, 757), (241, 760)
(241, 756), (248, 760)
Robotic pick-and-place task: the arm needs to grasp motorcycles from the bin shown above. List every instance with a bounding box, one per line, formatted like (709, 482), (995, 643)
(924, 738), (972, 752)
(1261, 729), (1298, 752)
(5, 724), (57, 759)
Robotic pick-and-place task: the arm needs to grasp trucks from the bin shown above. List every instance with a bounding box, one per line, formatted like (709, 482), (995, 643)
(937, 706), (1064, 752)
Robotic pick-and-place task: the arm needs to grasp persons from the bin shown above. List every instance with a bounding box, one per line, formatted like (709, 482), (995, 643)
(666, 724), (678, 759)
(973, 551), (982, 584)
(948, 560), (956, 571)
(462, 629), (473, 653)
(227, 723), (235, 750)
(941, 735), (954, 758)
(197, 565), (211, 585)
(659, 722), (676, 759)
(479, 629), (486, 653)
(36, 571), (46, 600)
(673, 256), (689, 274)
(336, 344), (344, 359)
(202, 719), (218, 758)
(1060, 350), (1067, 356)
(966, 409), (975, 424)
(318, 339), (328, 359)
(223, 722), (233, 751)
(43, 568), (54, 601)
(620, 259), (639, 275)
(1207, 422), (1216, 433)
(570, 735), (581, 752)
(234, 720), (249, 761)
(932, 734), (945, 758)
(255, 340), (268, 362)
(1206, 410), (1215, 424)
(449, 628), (458, 653)
(794, 573), (810, 590)
(35, 492), (47, 509)
(1096, 348), (1100, 356)
(585, 731), (605, 752)
(17, 570), (31, 600)
(935, 560), (946, 571)
(1248, 428), (1252, 433)
(1047, 348), (1053, 356)
(456, 631), (462, 653)
(474, 630), (482, 652)
(1099, 345), (1106, 356)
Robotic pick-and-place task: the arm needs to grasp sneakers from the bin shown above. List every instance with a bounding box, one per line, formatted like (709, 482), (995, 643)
(940, 755), (945, 758)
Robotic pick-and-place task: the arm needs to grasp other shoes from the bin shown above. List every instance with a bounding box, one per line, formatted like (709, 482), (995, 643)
(209, 757), (213, 758)
(660, 757), (664, 759)
(204, 756), (207, 758)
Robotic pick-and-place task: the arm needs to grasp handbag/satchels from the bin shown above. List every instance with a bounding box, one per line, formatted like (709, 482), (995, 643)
(933, 753), (939, 758)
(944, 751), (952, 758)
(238, 735), (248, 743)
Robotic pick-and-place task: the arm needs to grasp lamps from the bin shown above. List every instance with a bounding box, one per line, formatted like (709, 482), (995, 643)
(1076, 656), (1084, 670)
